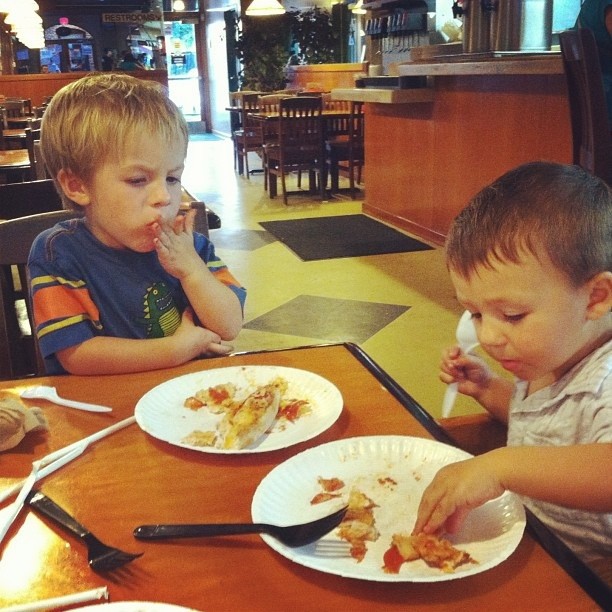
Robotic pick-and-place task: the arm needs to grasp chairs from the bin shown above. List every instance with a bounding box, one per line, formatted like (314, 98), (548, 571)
(323, 93), (365, 199)
(236, 93), (274, 178)
(258, 93), (304, 195)
(32, 106), (45, 118)
(27, 118), (41, 130)
(268, 97), (327, 204)
(24, 128), (52, 179)
(230, 91), (262, 170)
(320, 93), (353, 189)
(0, 203), (209, 377)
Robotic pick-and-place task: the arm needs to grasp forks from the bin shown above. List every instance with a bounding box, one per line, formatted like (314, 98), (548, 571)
(133, 503), (349, 549)
(25, 487), (144, 573)
(20, 385), (113, 414)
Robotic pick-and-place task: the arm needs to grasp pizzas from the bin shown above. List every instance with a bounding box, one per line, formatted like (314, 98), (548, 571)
(187, 379), (308, 451)
(313, 476), (470, 571)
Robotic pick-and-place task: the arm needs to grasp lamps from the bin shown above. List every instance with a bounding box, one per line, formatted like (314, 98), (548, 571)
(173, 0), (186, 12)
(245, 0), (286, 17)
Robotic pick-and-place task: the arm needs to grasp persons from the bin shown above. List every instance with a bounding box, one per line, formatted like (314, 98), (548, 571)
(39, 63), (50, 73)
(29, 73), (247, 375)
(412, 159), (612, 586)
(102, 47), (166, 72)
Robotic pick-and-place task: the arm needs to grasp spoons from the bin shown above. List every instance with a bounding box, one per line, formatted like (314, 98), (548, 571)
(440, 308), (484, 422)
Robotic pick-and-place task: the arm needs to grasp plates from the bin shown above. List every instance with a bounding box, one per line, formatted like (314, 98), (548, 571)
(249, 436), (530, 583)
(133, 365), (345, 455)
